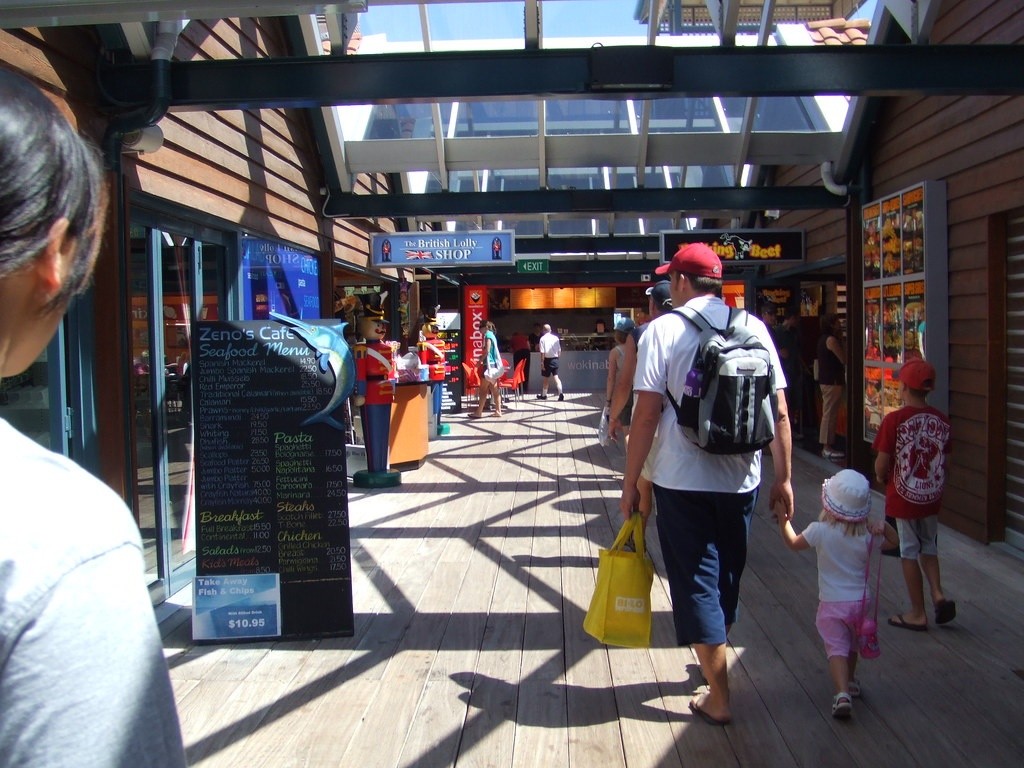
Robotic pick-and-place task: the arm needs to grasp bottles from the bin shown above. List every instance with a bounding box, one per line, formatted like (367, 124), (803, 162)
(382, 239), (392, 262)
(684, 358), (703, 397)
(492, 237), (502, 259)
(167, 392), (183, 407)
(138, 307), (144, 319)
(443, 321), (446, 328)
(801, 288), (813, 316)
(759, 288), (786, 323)
(176, 352), (181, 362)
(859, 619), (880, 658)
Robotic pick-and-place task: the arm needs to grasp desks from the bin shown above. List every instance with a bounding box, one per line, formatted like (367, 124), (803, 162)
(388, 380), (433, 472)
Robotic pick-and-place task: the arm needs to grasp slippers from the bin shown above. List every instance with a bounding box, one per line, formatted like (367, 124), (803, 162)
(888, 614), (927, 631)
(489, 411), (502, 417)
(934, 600), (957, 625)
(691, 685), (731, 725)
(468, 413), (482, 419)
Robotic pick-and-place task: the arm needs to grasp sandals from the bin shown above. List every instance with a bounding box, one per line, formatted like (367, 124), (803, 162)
(831, 693), (852, 717)
(846, 678), (861, 695)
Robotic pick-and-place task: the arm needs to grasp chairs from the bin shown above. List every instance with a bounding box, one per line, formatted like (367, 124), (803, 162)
(461, 358), (527, 411)
(166, 380), (179, 416)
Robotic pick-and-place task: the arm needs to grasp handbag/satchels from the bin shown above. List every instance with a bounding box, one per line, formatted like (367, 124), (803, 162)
(583, 512), (653, 647)
(598, 407), (611, 448)
(484, 363), (504, 381)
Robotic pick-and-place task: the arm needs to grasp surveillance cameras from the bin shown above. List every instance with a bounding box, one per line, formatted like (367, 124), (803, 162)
(764, 209), (779, 220)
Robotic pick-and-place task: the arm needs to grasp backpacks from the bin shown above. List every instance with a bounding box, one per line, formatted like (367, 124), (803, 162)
(667, 306), (775, 455)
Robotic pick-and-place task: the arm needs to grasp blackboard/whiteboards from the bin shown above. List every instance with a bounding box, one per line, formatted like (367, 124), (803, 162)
(189, 318), (354, 645)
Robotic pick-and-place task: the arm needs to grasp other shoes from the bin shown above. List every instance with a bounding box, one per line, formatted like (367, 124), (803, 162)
(558, 394), (563, 402)
(537, 394), (548, 401)
(822, 449), (846, 459)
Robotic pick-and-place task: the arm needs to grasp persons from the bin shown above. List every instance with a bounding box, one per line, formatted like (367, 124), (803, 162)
(815, 316), (845, 459)
(417, 306), (445, 424)
(0, 65), (184, 768)
(537, 324), (564, 401)
(774, 469), (901, 717)
(759, 305), (811, 439)
(529, 323), (543, 351)
(353, 293), (393, 473)
(869, 354), (959, 632)
(605, 281), (672, 545)
(619, 242), (795, 724)
(467, 320), (503, 417)
(507, 331), (530, 395)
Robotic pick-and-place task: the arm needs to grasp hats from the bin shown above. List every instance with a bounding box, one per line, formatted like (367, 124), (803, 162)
(655, 243), (722, 279)
(822, 469), (872, 520)
(613, 317), (634, 332)
(898, 358), (935, 392)
(645, 280), (674, 306)
